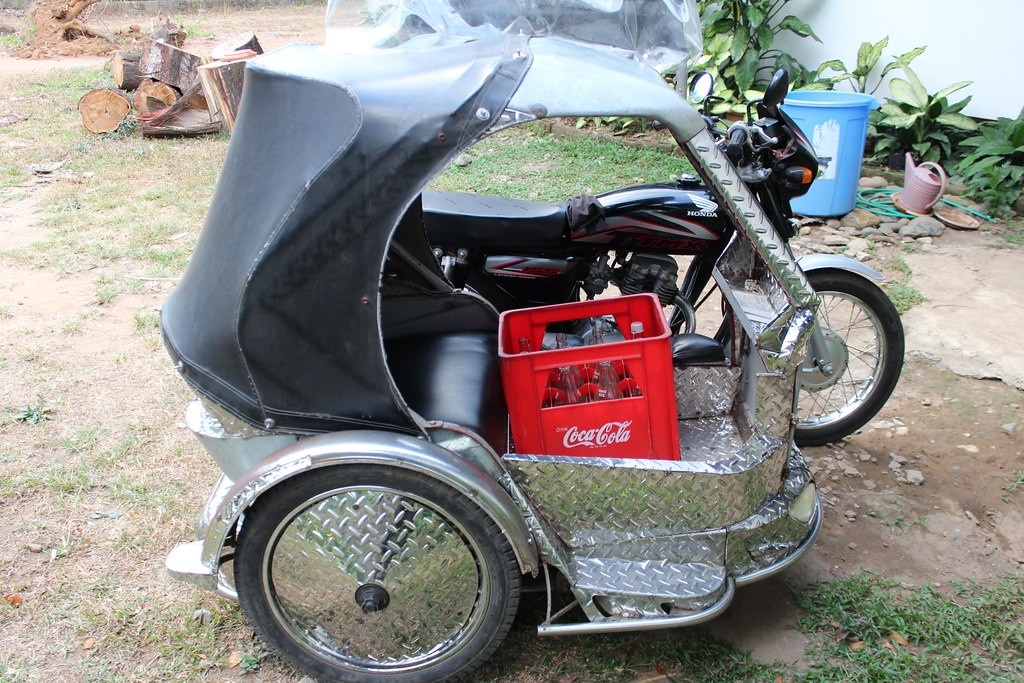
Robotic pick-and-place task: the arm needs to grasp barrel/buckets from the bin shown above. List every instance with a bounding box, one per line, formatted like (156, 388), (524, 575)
(776, 90), (880, 217)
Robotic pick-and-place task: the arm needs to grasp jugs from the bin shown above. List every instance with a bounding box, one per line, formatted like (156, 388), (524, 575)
(900, 152), (946, 214)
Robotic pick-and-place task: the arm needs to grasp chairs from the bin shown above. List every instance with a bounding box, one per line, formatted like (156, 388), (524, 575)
(383, 333), (506, 459)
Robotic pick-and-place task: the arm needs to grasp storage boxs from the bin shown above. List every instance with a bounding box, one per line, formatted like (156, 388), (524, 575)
(496, 292), (684, 462)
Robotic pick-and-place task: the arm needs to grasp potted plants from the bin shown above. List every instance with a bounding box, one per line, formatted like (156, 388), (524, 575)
(954, 106), (1024, 217)
(687, 0), (824, 123)
(878, 56), (979, 172)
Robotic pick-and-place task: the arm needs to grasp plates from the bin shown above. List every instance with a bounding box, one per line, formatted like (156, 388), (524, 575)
(933, 209), (980, 229)
(891, 193), (934, 217)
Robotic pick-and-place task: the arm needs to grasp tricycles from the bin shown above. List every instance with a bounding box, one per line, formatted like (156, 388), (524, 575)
(155, 33), (821, 683)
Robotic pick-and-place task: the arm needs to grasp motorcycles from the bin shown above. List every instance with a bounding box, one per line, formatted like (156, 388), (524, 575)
(422, 69), (905, 447)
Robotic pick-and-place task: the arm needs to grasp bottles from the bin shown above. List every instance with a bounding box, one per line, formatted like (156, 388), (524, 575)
(518, 322), (644, 408)
